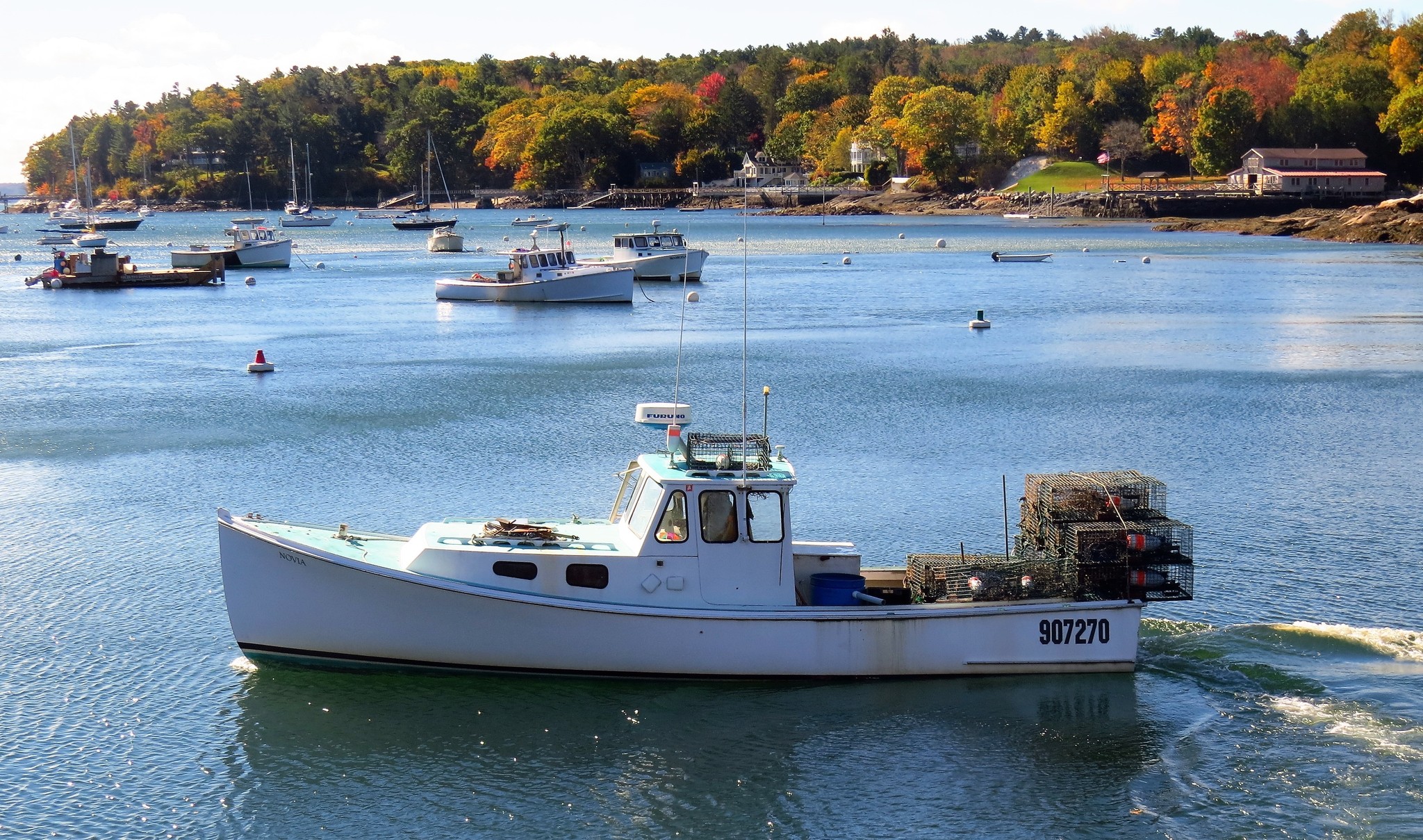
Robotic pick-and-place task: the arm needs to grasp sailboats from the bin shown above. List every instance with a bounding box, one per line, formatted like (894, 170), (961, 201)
(77, 158), (108, 247)
(403, 164), (431, 217)
(137, 146), (155, 216)
(103, 178), (127, 214)
(231, 160), (265, 225)
(61, 124), (144, 231)
(281, 138), (313, 215)
(393, 131), (458, 230)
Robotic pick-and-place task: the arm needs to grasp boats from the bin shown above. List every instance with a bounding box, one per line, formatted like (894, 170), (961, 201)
(24, 248), (224, 285)
(512, 216), (553, 226)
(45, 210), (100, 225)
(575, 220), (709, 281)
(535, 224), (570, 231)
(354, 212), (407, 218)
(37, 235), (77, 245)
(280, 213), (338, 226)
(0, 226), (9, 233)
(436, 234), (635, 303)
(1003, 214), (1033, 218)
(427, 225), (464, 252)
(992, 251), (1052, 262)
(171, 228), (293, 268)
(216, 434), (1144, 684)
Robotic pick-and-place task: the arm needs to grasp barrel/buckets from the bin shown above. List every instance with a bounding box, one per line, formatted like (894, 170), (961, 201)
(123, 263), (134, 274)
(809, 572), (866, 606)
